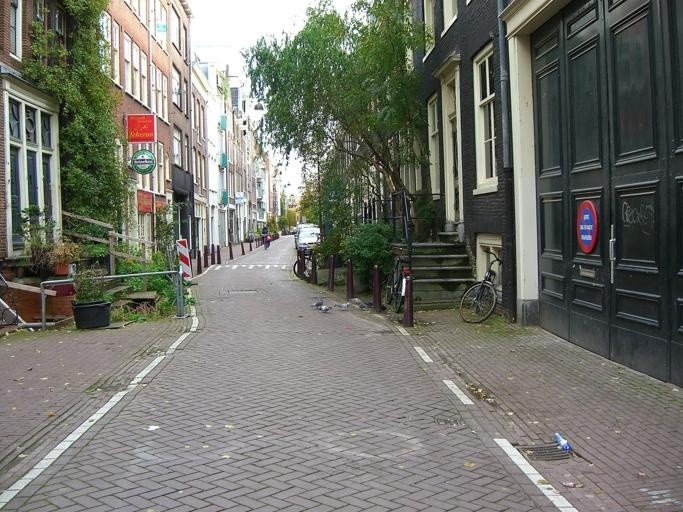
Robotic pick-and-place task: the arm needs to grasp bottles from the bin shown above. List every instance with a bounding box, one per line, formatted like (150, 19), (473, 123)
(554, 432), (570, 451)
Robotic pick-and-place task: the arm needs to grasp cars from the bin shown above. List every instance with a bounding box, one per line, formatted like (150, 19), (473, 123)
(290, 224), (322, 255)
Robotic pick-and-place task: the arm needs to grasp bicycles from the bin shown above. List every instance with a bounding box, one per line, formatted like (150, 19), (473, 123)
(260, 233), (269, 250)
(383, 246), (413, 314)
(458, 249), (505, 324)
(291, 231), (322, 282)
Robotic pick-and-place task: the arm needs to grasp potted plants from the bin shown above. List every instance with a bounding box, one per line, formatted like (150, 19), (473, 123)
(70, 270), (112, 330)
(16, 205), (79, 280)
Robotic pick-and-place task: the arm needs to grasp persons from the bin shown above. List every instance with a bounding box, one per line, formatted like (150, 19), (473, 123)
(259, 223), (271, 250)
